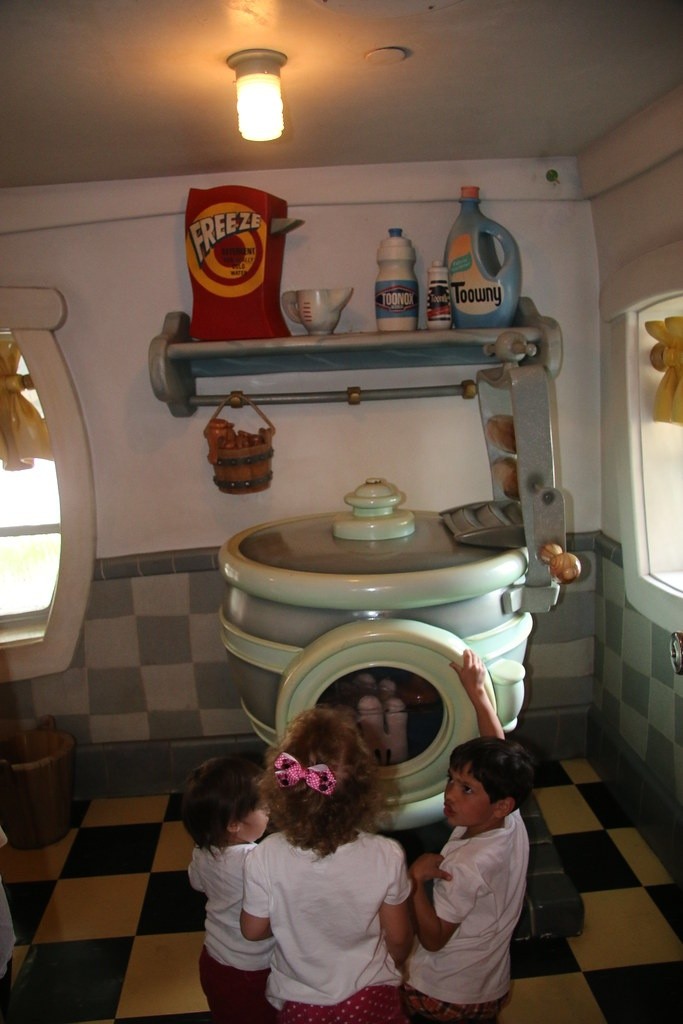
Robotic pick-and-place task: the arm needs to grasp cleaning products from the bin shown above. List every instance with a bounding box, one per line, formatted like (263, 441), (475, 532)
(426, 260), (452, 330)
(375, 228), (419, 330)
(444, 186), (521, 329)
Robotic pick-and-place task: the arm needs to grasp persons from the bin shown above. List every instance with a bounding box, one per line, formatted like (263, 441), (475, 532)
(180, 759), (278, 1024)
(402, 650), (534, 1024)
(0, 825), (16, 1024)
(239, 706), (413, 1024)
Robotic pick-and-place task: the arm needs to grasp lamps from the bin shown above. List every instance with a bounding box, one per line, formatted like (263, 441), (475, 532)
(226, 48), (288, 142)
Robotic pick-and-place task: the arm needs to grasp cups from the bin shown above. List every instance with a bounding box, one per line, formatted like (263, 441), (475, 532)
(282, 287), (354, 336)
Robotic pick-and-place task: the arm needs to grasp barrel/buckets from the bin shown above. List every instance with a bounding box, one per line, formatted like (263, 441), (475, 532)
(0, 714), (78, 851)
(204, 393), (276, 494)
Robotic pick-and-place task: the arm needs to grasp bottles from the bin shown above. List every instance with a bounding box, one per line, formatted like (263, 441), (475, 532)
(445, 186), (521, 328)
(374, 228), (419, 332)
(425, 260), (452, 330)
